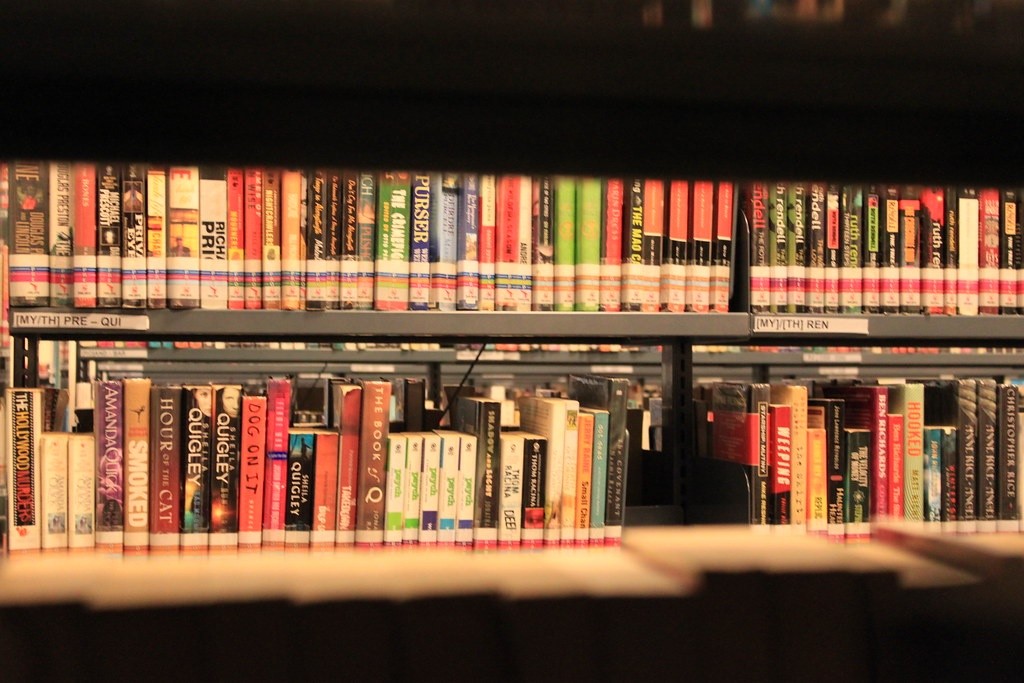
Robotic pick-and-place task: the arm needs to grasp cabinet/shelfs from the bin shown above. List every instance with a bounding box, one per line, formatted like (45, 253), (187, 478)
(0, 0), (1024, 683)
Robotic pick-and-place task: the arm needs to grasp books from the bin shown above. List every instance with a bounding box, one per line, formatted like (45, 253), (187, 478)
(0, 161), (737, 313)
(694, 345), (1024, 547)
(0, 334), (664, 564)
(740, 180), (1024, 317)
